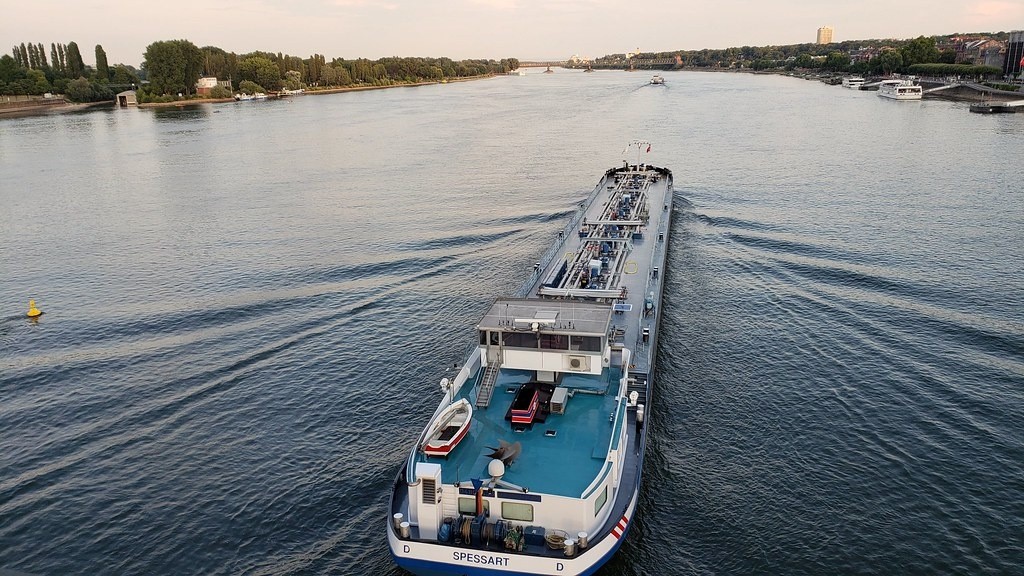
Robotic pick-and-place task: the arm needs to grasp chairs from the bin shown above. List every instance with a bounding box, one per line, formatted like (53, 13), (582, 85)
(615, 325), (628, 340)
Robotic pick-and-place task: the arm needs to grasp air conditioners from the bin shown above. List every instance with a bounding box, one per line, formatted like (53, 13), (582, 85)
(569, 356), (586, 372)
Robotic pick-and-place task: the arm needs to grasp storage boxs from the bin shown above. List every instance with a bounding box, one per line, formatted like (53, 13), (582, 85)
(524, 525), (545, 547)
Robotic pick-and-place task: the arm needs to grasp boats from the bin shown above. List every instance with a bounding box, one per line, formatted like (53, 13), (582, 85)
(235, 86), (305, 102)
(651, 75), (664, 84)
(877, 78), (923, 101)
(842, 78), (866, 89)
(421, 397), (472, 460)
(970, 100), (1017, 113)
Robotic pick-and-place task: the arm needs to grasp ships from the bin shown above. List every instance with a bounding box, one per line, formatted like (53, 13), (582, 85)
(385, 139), (673, 573)
